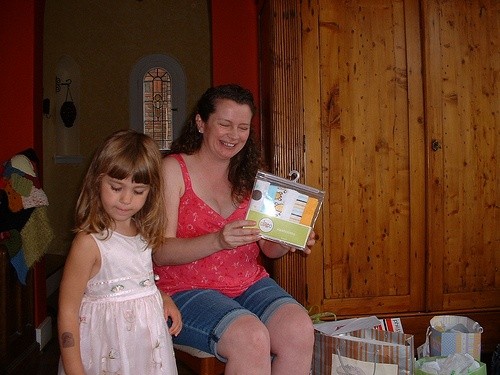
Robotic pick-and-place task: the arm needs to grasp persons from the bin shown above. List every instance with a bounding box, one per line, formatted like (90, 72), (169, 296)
(152, 82), (316, 375)
(58, 130), (182, 375)
